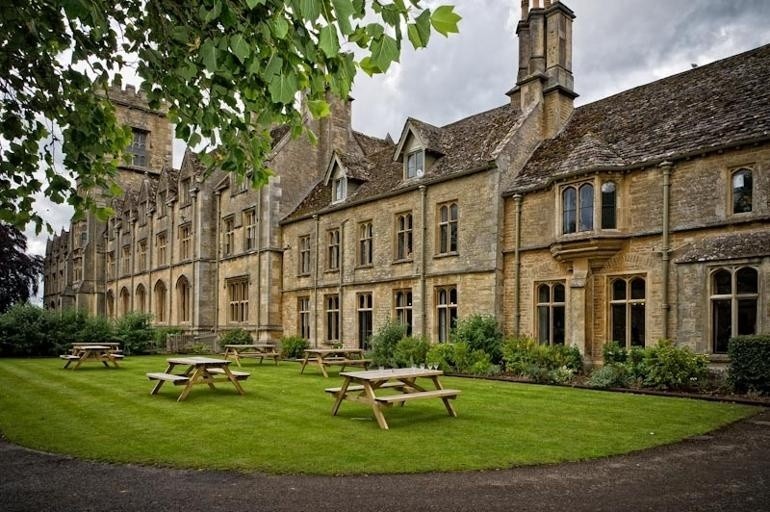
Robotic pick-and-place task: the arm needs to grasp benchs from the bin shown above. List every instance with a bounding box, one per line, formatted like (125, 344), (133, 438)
(56, 340), (132, 374)
(140, 343), (284, 405)
(293, 346), (463, 431)
